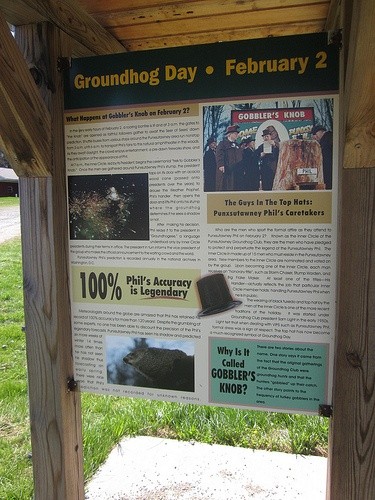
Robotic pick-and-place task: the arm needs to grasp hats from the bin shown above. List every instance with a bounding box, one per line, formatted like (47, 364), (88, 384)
(261, 129), (272, 137)
(308, 125), (327, 134)
(224, 126), (240, 135)
(206, 137), (217, 146)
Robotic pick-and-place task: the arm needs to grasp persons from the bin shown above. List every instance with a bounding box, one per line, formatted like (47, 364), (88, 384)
(204, 122), (335, 191)
